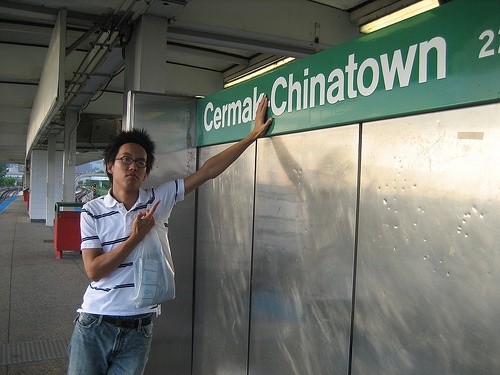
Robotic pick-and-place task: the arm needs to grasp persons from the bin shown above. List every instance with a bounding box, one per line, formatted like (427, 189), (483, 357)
(68, 95), (274, 375)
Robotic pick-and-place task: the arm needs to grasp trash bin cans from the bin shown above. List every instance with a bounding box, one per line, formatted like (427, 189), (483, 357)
(24, 187), (29, 201)
(54, 202), (87, 258)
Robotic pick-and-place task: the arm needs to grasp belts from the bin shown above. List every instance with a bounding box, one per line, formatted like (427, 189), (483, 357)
(89, 314), (154, 328)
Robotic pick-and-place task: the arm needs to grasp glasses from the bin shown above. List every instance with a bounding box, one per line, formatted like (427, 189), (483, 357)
(113, 156), (146, 168)
